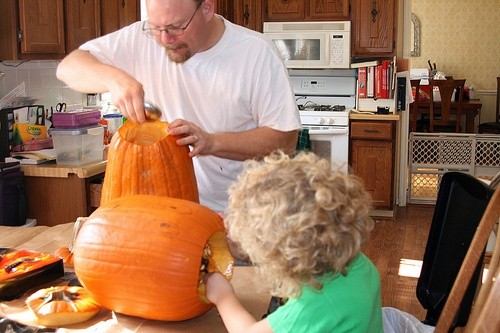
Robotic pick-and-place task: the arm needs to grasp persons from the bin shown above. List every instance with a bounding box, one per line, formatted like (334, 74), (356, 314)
(56, 0), (302, 218)
(204, 149), (383, 333)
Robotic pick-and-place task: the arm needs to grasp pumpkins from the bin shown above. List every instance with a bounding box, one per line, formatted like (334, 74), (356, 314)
(72, 194), (235, 321)
(99, 117), (201, 205)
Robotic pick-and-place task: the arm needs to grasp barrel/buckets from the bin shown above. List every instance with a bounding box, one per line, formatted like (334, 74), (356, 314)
(103, 114), (123, 134)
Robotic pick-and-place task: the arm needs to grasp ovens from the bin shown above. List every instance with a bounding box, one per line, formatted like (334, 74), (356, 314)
(298, 126), (348, 176)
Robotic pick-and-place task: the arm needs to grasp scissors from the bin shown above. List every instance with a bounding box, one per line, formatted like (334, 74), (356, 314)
(56, 103), (66, 112)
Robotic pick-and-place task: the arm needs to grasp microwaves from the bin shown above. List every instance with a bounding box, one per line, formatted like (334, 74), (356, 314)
(262, 21), (351, 69)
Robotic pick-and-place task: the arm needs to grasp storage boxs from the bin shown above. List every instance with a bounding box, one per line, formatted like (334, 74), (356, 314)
(47, 124), (106, 166)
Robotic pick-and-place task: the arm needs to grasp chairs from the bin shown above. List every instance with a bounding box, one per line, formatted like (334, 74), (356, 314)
(413, 166), (500, 333)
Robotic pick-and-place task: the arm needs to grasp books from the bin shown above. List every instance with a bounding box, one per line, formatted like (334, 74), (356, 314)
(358, 55), (397, 99)
(5, 149), (56, 164)
(351, 59), (380, 68)
(397, 70), (413, 112)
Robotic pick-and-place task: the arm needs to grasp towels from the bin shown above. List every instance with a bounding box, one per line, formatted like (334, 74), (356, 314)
(296, 128), (311, 151)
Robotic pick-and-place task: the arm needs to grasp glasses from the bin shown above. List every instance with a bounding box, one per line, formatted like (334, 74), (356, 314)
(142, 0), (204, 38)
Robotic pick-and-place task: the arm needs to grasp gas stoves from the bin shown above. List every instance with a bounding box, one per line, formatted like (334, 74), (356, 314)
(287, 69), (357, 126)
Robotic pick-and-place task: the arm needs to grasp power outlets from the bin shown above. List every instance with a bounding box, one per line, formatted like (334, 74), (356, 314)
(86, 91), (98, 107)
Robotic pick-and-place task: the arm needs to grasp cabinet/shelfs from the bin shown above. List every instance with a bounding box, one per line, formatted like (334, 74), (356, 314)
(0, 0), (404, 62)
(20, 161), (110, 227)
(348, 111), (404, 221)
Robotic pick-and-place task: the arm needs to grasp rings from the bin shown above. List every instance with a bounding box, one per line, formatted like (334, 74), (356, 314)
(194, 135), (197, 140)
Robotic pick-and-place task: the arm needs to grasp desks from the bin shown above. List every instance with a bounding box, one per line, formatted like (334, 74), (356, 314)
(0, 222), (274, 333)
